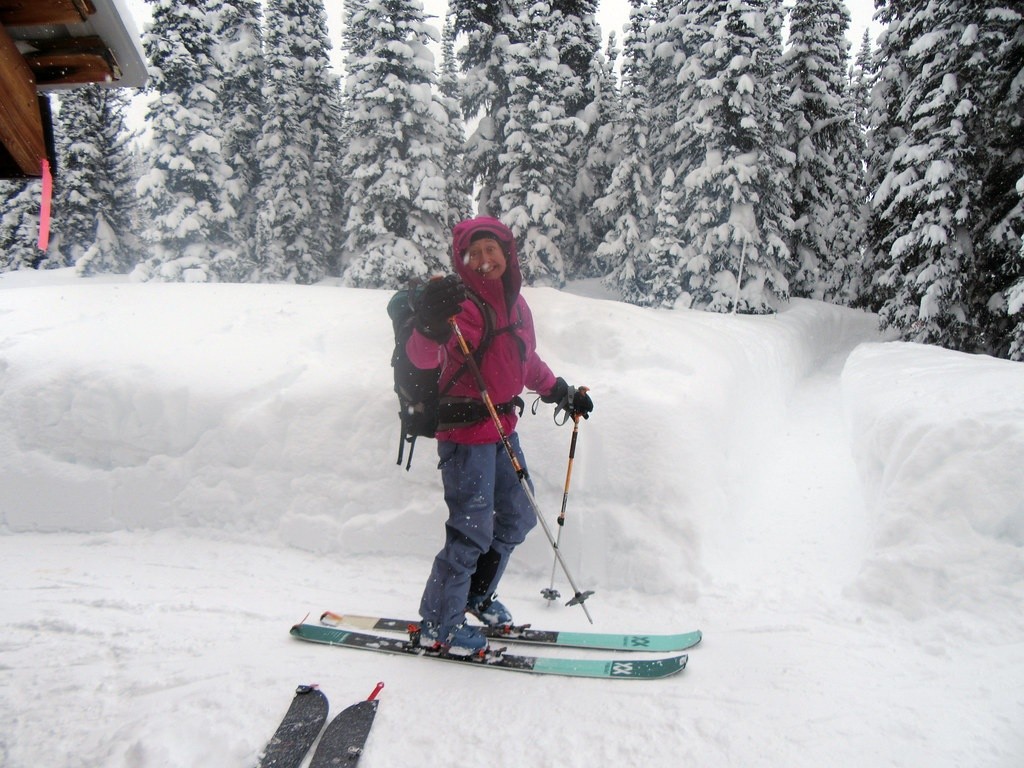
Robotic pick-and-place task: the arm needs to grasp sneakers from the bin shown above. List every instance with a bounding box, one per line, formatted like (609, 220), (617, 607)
(420, 620), (489, 656)
(466, 593), (513, 629)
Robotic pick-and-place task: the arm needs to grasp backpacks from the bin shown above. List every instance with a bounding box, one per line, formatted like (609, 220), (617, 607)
(387, 273), (525, 472)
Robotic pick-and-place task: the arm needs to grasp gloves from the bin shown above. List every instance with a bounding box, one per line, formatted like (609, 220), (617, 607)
(414, 273), (467, 344)
(541, 377), (593, 423)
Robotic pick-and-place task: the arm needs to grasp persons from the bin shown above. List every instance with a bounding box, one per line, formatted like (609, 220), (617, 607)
(405, 217), (593, 656)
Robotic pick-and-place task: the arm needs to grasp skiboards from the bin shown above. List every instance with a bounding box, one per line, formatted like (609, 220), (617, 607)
(256, 682), (388, 768)
(289, 611), (702, 680)
